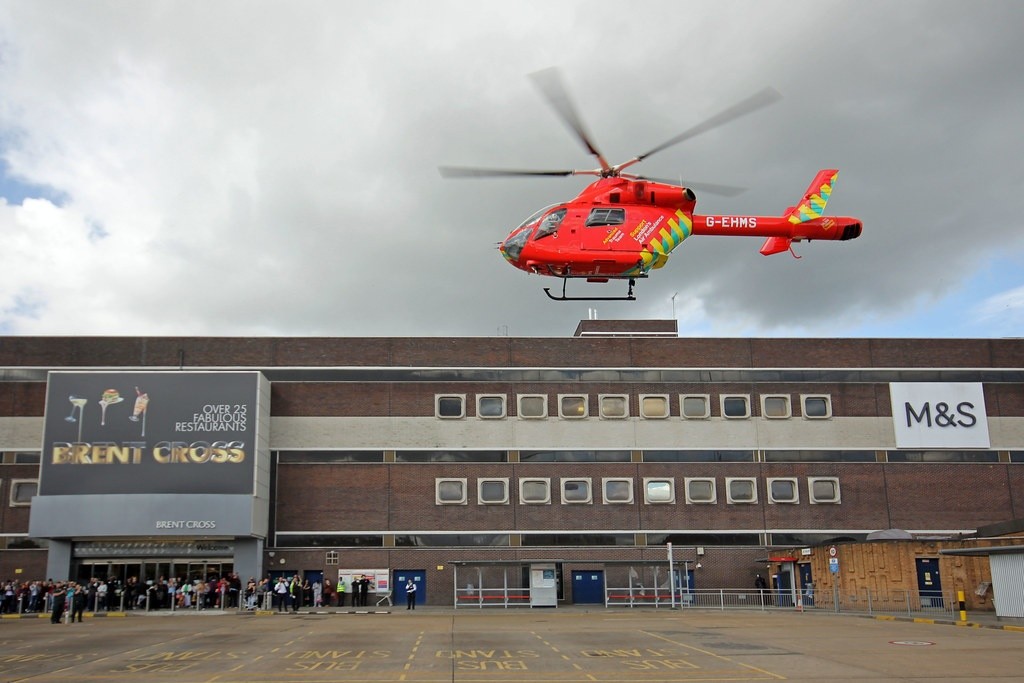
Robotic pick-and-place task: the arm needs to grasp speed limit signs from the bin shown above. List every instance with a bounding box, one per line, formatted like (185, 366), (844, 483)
(829, 547), (837, 557)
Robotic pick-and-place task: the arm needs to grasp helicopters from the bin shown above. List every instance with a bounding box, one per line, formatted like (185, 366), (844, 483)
(432, 66), (866, 302)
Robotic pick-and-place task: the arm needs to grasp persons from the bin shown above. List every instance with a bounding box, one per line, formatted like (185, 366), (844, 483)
(405, 579), (417, 610)
(0, 571), (371, 624)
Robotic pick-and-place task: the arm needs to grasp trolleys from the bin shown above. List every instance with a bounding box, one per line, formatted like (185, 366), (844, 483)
(376, 588), (394, 607)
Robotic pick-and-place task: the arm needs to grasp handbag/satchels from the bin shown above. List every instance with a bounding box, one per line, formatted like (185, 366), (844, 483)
(317, 595), (323, 602)
(184, 592), (191, 606)
